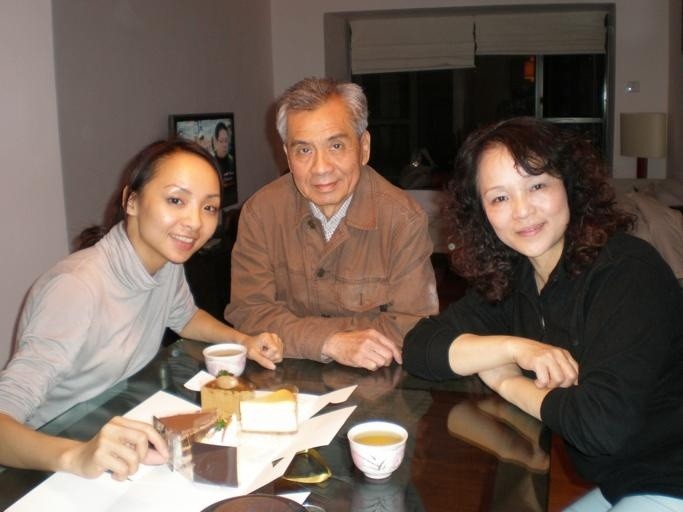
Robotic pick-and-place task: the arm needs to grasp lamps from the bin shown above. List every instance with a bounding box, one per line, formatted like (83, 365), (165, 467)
(619, 112), (667, 180)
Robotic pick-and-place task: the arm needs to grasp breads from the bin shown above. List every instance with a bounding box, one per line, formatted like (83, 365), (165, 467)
(200, 370), (256, 421)
(192, 440), (257, 488)
(152, 409), (232, 473)
(240, 387), (300, 434)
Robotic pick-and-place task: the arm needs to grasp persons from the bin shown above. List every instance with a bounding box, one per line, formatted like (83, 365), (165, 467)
(435, 386), (552, 479)
(401, 148), (438, 190)
(225, 77), (439, 373)
(1, 137), (283, 483)
(245, 357), (431, 512)
(196, 124), (238, 209)
(403, 119), (682, 512)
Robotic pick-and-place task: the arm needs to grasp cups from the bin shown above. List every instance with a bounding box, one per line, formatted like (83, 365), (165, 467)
(347, 421), (409, 481)
(202, 343), (247, 378)
